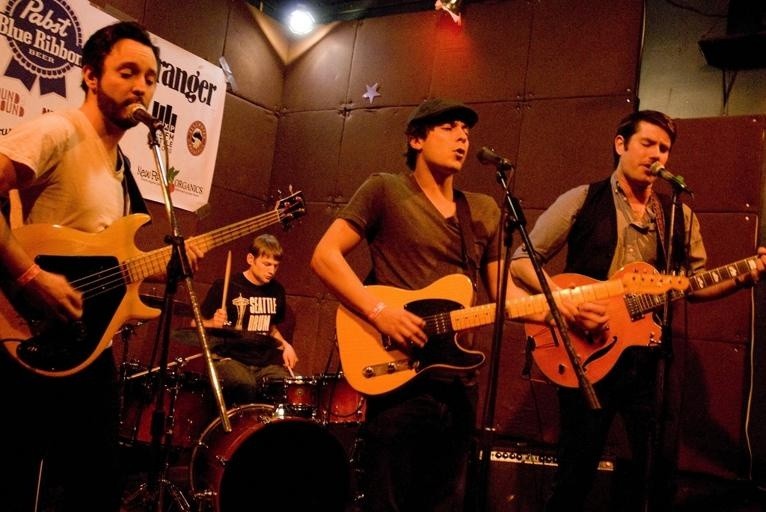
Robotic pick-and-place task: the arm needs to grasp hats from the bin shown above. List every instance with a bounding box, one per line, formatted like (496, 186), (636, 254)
(406, 99), (478, 130)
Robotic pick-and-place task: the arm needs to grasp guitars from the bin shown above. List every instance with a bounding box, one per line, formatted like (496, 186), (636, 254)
(334, 272), (689, 397)
(0, 185), (307, 376)
(525, 256), (765, 388)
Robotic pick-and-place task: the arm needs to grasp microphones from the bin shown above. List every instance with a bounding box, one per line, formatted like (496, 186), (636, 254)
(649, 160), (695, 196)
(476, 146), (514, 172)
(125, 102), (165, 131)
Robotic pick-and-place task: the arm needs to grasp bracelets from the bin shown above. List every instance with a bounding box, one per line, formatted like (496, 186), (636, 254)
(16, 264), (41, 288)
(366, 302), (385, 321)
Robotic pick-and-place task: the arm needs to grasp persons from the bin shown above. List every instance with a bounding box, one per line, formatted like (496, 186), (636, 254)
(509, 111), (766, 512)
(190, 233), (299, 405)
(311, 98), (556, 512)
(0, 20), (161, 512)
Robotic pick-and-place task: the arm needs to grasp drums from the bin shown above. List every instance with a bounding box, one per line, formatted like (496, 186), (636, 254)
(118, 363), (210, 452)
(283, 373), (366, 422)
(189, 405), (357, 512)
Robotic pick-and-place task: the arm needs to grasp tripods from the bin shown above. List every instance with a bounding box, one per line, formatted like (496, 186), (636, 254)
(118, 353), (193, 512)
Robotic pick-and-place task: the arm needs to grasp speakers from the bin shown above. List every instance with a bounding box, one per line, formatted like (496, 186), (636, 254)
(485, 449), (615, 512)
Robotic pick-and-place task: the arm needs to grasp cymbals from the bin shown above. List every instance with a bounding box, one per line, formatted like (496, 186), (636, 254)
(140, 292), (197, 316)
(174, 328), (283, 350)
